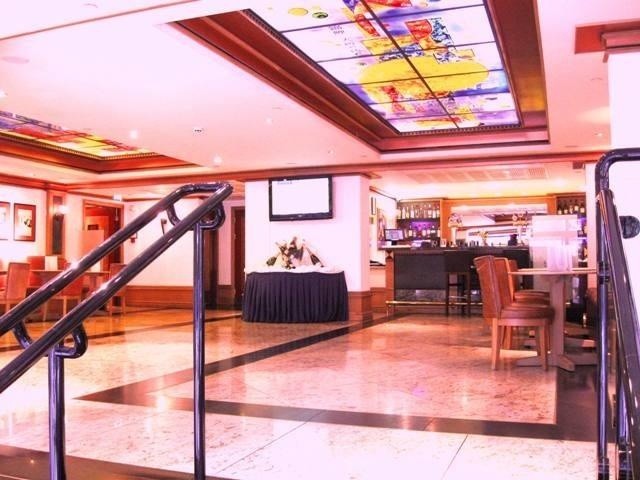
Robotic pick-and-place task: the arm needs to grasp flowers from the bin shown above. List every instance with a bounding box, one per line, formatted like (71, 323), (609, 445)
(448, 213), (462, 228)
(478, 231), (488, 239)
(512, 212), (527, 226)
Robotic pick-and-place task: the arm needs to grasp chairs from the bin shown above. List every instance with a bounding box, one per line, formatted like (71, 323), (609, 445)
(0, 257), (127, 322)
(473, 254), (555, 373)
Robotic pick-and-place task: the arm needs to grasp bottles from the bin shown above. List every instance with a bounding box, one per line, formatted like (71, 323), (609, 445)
(408, 222), (440, 239)
(397, 202), (440, 219)
(557, 199), (585, 214)
(266, 236), (323, 267)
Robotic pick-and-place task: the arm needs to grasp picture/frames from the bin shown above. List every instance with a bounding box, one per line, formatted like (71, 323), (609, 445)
(0, 201), (10, 240)
(13, 203), (36, 241)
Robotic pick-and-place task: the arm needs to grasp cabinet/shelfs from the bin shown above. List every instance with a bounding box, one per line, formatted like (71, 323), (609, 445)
(548, 192), (588, 267)
(397, 198), (443, 247)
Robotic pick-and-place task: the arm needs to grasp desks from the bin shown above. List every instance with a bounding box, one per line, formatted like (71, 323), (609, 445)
(242, 267), (349, 323)
(508, 267), (599, 372)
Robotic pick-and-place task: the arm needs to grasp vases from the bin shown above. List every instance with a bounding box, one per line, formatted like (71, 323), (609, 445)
(517, 225), (522, 244)
(451, 226), (458, 247)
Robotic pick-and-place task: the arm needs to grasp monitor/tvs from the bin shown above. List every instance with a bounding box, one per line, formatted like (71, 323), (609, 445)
(383, 228), (405, 246)
(269, 176), (334, 222)
